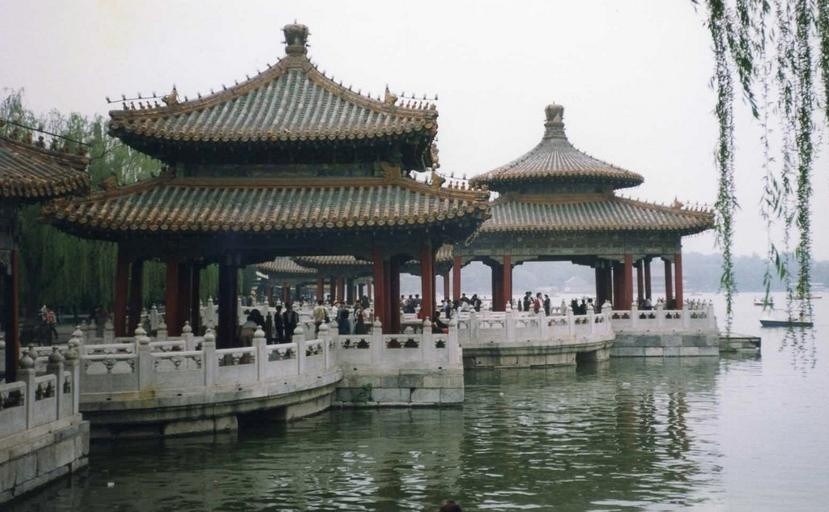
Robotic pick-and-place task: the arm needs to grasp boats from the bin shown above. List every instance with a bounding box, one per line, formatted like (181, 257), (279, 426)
(753, 297), (774, 306)
(760, 320), (814, 329)
(786, 296), (823, 300)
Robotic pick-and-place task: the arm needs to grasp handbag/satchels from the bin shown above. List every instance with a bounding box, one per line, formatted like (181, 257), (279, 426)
(325, 316), (329, 323)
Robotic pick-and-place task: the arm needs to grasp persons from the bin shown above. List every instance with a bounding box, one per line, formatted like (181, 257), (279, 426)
(44, 306), (58, 339)
